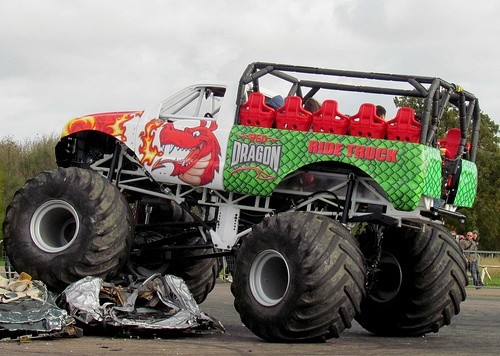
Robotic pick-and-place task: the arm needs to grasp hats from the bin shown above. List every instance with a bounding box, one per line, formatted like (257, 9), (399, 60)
(472, 230), (480, 235)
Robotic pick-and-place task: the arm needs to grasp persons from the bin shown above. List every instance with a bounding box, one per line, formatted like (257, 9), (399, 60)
(459, 231), (481, 287)
(375, 106), (387, 124)
(302, 97), (324, 118)
(470, 230), (484, 285)
(450, 231), (457, 239)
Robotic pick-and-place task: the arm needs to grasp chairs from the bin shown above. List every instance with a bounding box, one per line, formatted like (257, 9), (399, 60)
(275, 96), (313, 131)
(348, 103), (387, 139)
(437, 128), (472, 167)
(313, 100), (349, 135)
(386, 107), (422, 143)
(239, 92), (276, 129)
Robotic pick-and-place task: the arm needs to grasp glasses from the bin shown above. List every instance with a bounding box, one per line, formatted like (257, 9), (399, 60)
(467, 235), (472, 238)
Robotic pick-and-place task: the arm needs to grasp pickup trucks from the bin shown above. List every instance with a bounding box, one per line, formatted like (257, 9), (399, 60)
(1, 62), (480, 341)
